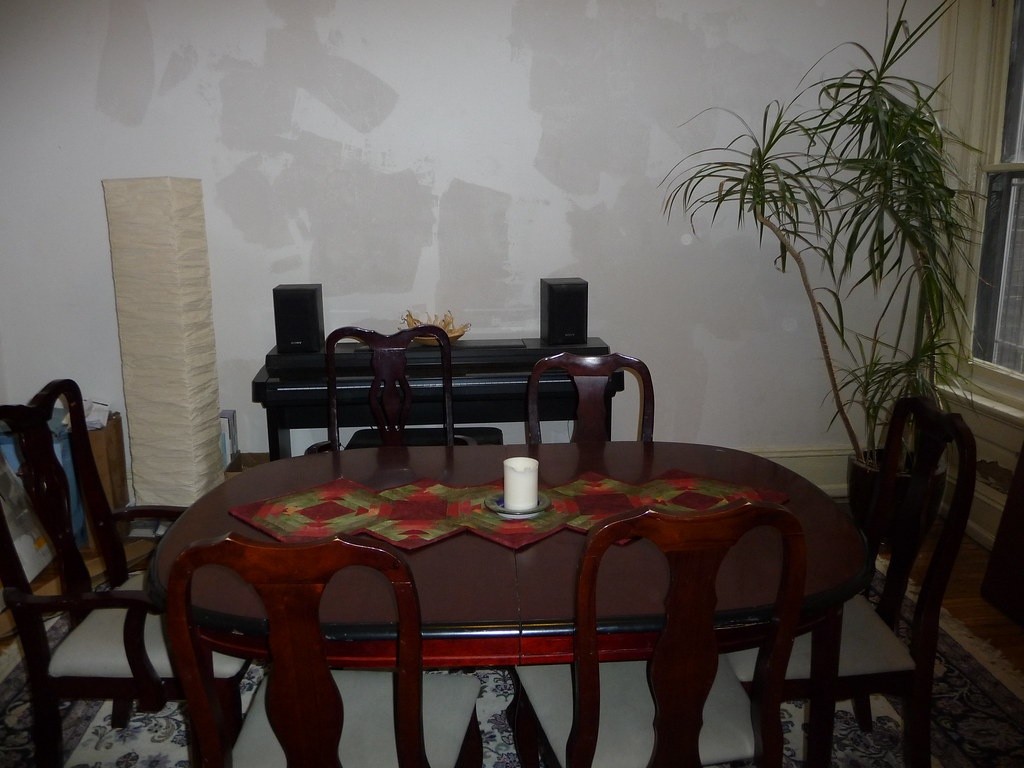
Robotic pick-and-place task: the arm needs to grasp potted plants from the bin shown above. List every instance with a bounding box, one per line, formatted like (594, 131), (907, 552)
(656, 0), (993, 547)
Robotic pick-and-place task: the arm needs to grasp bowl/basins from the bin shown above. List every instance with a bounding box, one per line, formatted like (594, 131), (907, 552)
(415, 329), (465, 347)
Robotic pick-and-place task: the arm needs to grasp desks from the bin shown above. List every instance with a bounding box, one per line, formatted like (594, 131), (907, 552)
(146, 441), (871, 768)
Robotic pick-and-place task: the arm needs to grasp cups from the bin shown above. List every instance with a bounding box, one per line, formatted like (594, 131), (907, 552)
(502, 457), (539, 510)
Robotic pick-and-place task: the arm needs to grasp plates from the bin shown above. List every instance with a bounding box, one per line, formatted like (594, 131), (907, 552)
(484, 492), (550, 519)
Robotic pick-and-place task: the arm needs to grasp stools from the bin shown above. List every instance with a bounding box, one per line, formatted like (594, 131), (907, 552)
(344, 428), (503, 450)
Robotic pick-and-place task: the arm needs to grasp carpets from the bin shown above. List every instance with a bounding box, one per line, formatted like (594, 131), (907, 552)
(0, 555), (1024, 768)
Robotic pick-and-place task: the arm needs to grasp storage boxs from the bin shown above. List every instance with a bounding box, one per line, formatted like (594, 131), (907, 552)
(88, 415), (130, 510)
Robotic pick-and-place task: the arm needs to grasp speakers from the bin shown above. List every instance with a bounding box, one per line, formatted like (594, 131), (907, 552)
(541, 277), (588, 345)
(273, 284), (326, 354)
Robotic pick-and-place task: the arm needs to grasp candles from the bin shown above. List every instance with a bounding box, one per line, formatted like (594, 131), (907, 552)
(502, 457), (539, 510)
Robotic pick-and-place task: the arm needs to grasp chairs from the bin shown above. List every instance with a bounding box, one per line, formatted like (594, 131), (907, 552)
(506, 498), (809, 768)
(163, 529), (483, 768)
(524, 350), (655, 444)
(0, 378), (254, 768)
(727, 394), (978, 768)
(304, 324), (477, 456)
